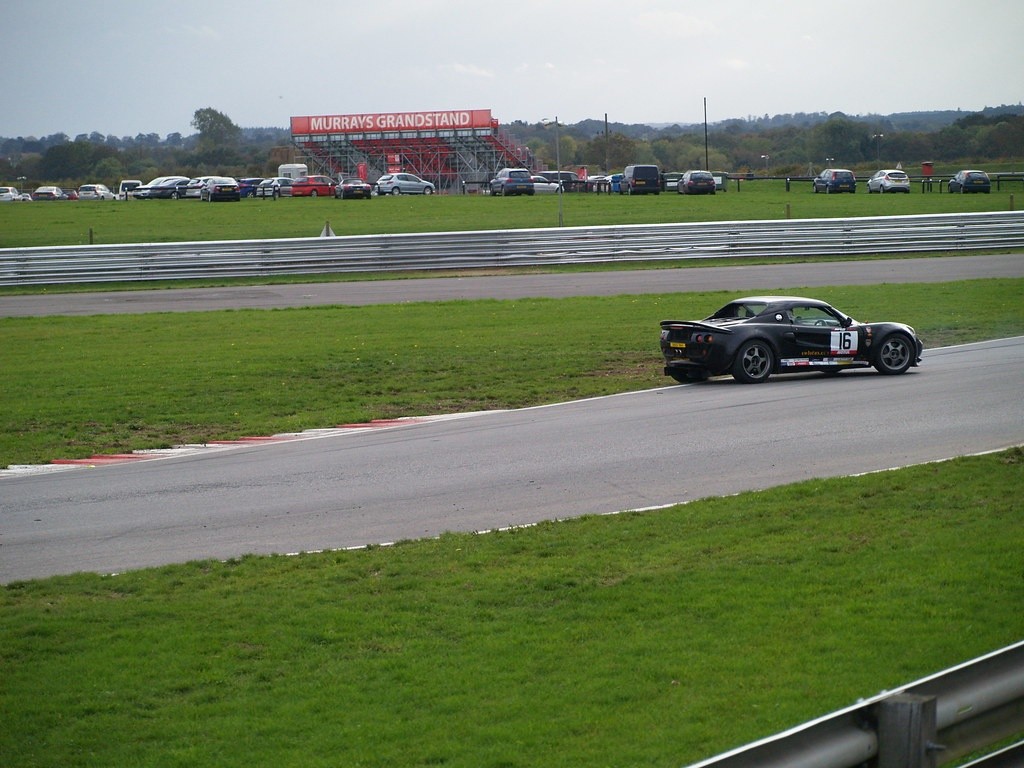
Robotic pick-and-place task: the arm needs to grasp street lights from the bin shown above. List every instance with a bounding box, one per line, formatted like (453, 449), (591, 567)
(872, 131), (883, 170)
(826, 152), (835, 168)
(16, 175), (26, 192)
(760, 154), (769, 173)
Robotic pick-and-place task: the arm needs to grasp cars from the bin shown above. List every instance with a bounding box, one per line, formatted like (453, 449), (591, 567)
(1, 176), (294, 203)
(677, 170), (715, 194)
(812, 168), (857, 194)
(334, 178), (371, 199)
(489, 167), (534, 196)
(375, 172), (435, 196)
(866, 169), (910, 193)
(530, 171), (622, 193)
(291, 175), (337, 197)
(947, 169), (991, 193)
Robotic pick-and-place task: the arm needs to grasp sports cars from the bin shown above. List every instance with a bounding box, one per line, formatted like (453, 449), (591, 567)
(658, 296), (924, 384)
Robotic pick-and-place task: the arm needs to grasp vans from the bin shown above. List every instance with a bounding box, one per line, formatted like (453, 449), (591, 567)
(618, 165), (661, 195)
